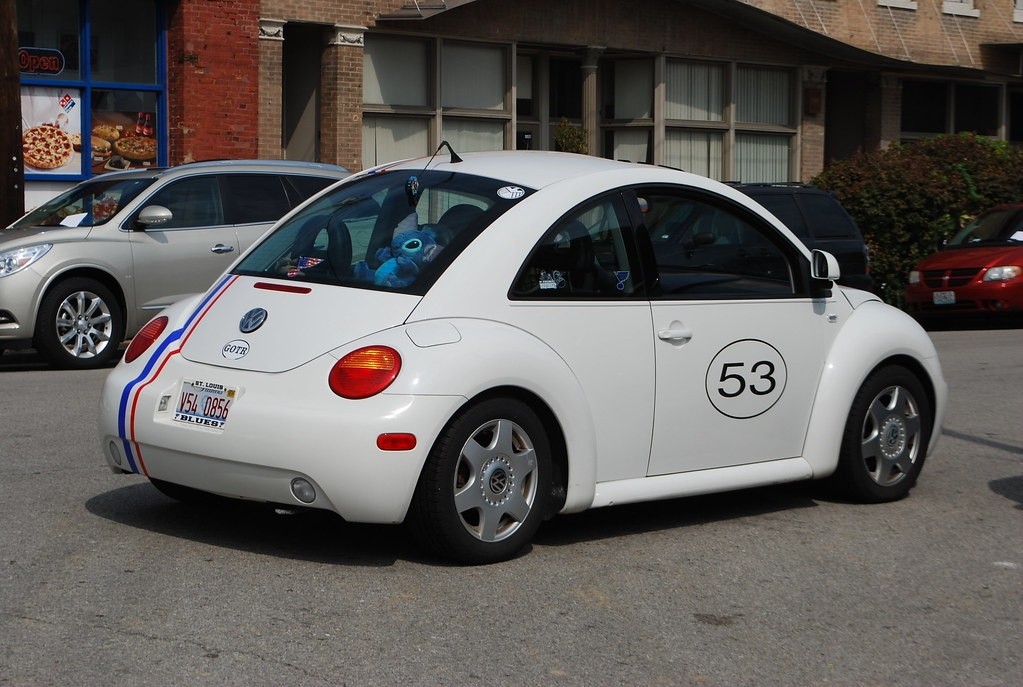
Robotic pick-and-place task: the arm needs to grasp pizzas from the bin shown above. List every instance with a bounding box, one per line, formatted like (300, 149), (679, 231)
(92, 196), (119, 224)
(23, 126), (73, 168)
(113, 137), (157, 159)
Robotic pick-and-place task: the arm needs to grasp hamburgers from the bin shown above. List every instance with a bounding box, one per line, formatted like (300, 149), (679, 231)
(91, 136), (111, 163)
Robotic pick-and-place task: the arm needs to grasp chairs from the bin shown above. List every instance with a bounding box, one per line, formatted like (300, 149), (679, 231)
(533, 219), (600, 298)
(437, 204), (494, 256)
(295, 214), (354, 278)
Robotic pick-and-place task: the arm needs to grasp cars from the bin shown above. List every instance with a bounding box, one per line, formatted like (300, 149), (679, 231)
(905, 202), (1023, 330)
(0, 160), (386, 369)
(97, 147), (948, 562)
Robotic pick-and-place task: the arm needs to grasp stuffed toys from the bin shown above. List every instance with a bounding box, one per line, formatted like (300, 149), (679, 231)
(354, 212), (444, 289)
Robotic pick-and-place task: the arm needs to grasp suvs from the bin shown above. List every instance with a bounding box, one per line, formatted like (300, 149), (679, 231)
(586, 177), (874, 291)
